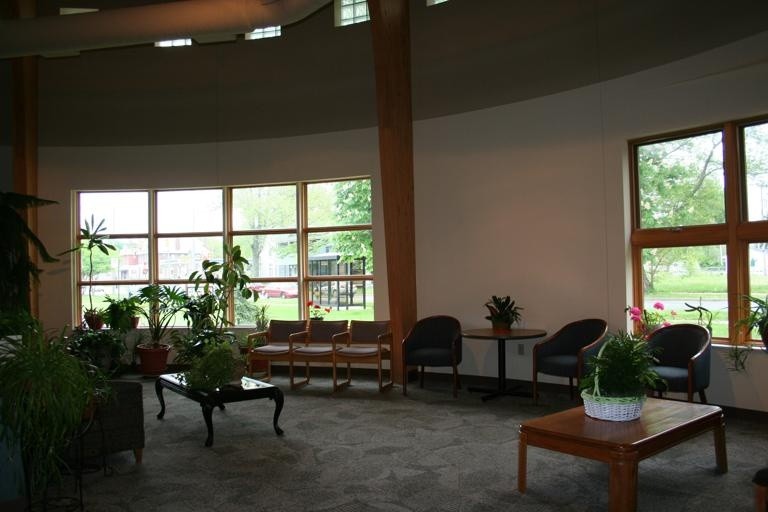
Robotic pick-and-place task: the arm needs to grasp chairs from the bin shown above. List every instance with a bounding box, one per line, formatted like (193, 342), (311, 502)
(246, 319), (395, 393)
(532, 318), (609, 406)
(644, 324), (711, 402)
(401, 315), (462, 398)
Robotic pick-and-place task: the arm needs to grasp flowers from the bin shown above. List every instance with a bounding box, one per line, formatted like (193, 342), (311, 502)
(623, 302), (677, 342)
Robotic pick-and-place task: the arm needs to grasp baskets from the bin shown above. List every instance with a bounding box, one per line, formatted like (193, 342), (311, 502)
(581, 339), (646, 421)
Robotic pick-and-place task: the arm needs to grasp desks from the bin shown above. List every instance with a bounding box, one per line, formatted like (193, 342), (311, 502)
(154, 372), (283, 448)
(517, 396), (729, 511)
(460, 328), (547, 402)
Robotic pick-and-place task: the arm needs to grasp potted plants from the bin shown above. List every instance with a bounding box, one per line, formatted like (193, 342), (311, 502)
(718, 292), (767, 375)
(0, 185), (268, 512)
(483, 295), (523, 333)
(580, 328), (666, 422)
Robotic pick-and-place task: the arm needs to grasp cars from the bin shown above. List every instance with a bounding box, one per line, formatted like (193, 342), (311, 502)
(263, 283), (299, 299)
(352, 269), (374, 287)
(241, 283), (264, 294)
(312, 280), (357, 299)
